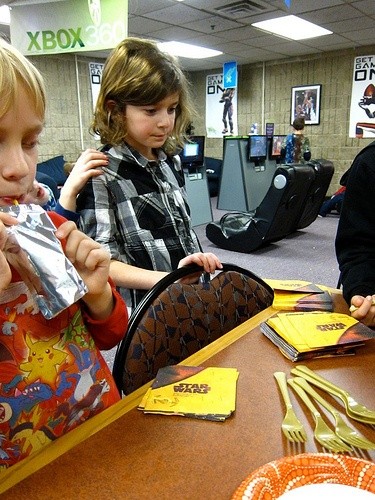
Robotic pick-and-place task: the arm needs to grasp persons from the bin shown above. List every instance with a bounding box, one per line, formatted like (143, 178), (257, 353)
(294, 91), (316, 123)
(0, 38), (130, 481)
(318, 185), (347, 218)
(284, 119), (312, 165)
(24, 148), (109, 226)
(334, 142), (375, 330)
(219, 88), (235, 135)
(75, 36), (224, 315)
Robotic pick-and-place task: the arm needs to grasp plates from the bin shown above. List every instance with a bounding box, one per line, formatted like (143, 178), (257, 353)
(231, 453), (375, 500)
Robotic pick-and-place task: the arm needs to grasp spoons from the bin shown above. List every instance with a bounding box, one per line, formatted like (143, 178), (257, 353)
(289, 364), (374, 425)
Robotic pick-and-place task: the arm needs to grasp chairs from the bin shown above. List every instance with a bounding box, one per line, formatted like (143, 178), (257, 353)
(206, 163), (316, 256)
(112, 259), (277, 402)
(295, 159), (334, 230)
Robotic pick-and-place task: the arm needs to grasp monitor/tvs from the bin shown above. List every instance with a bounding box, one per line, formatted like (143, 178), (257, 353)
(270, 135), (288, 160)
(178, 136), (205, 168)
(249, 135), (268, 162)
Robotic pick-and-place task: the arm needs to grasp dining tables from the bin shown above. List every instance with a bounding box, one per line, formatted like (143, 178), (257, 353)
(0, 284), (375, 500)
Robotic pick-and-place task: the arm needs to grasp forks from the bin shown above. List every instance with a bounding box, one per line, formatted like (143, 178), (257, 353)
(273, 371), (375, 459)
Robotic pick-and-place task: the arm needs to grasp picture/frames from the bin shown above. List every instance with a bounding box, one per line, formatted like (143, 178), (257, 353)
(290, 84), (322, 126)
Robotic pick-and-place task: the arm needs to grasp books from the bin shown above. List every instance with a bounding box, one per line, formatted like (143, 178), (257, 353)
(136, 279), (375, 423)
